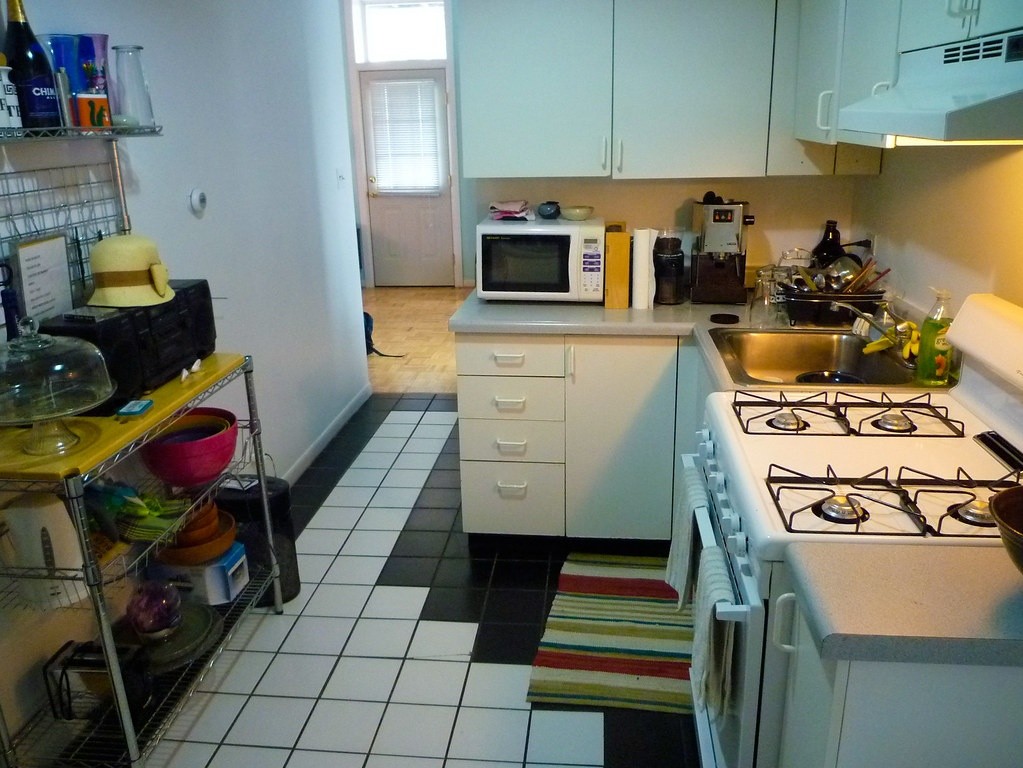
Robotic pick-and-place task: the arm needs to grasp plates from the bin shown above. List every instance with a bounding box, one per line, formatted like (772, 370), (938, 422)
(111, 600), (224, 673)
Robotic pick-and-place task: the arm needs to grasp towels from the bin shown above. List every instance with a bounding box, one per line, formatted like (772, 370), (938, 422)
(664, 465), (710, 613)
(691, 545), (737, 733)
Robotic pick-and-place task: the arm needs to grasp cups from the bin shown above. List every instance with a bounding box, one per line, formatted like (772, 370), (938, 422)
(749, 265), (792, 322)
(66, 94), (112, 136)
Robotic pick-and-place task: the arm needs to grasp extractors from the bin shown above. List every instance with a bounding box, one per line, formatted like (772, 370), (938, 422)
(836, 29), (1023, 141)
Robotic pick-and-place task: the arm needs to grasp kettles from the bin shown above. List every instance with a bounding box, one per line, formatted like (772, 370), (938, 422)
(0, 492), (91, 608)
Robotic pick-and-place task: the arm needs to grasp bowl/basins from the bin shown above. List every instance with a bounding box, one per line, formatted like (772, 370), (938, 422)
(142, 407), (239, 487)
(796, 370), (866, 384)
(988, 485), (1023, 575)
(560, 205), (594, 221)
(151, 496), (236, 563)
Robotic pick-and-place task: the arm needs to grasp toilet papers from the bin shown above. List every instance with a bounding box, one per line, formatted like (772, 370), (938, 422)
(632, 227), (660, 311)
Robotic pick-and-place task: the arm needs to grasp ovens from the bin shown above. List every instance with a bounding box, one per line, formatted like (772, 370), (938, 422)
(681, 452), (767, 768)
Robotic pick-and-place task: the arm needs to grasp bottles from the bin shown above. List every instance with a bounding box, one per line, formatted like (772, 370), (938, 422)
(810, 221), (846, 269)
(915, 289), (953, 387)
(653, 237), (686, 304)
(36, 33), (118, 115)
(0, 66), (24, 136)
(0, 0), (61, 138)
(112, 45), (155, 132)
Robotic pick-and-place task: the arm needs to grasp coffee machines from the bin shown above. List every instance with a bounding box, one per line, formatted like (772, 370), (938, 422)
(689, 198), (755, 306)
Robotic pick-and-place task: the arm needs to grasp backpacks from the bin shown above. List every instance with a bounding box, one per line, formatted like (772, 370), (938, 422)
(364, 311), (375, 354)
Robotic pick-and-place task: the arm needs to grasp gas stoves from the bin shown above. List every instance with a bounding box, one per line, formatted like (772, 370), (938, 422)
(697, 294), (1023, 597)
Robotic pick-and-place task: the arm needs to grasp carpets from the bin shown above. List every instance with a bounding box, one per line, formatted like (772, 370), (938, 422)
(524, 552), (699, 713)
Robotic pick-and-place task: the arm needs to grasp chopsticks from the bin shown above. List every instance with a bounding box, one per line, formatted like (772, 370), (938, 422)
(841, 257), (892, 294)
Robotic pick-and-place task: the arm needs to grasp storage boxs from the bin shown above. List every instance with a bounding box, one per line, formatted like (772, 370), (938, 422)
(36, 278), (220, 417)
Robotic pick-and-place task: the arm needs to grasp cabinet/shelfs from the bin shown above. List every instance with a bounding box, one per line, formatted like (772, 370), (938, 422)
(455, 1), (905, 179)
(457, 331), (676, 543)
(0, 353), (284, 768)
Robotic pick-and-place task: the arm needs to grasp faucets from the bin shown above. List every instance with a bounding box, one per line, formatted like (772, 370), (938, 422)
(829, 299), (918, 370)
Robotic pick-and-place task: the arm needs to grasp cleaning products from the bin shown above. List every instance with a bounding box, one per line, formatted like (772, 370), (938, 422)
(915, 285), (956, 386)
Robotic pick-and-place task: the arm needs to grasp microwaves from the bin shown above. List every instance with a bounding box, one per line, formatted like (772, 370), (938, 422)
(475, 216), (606, 302)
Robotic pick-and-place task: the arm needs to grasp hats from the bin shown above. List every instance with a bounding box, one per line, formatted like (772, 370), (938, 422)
(87, 235), (175, 307)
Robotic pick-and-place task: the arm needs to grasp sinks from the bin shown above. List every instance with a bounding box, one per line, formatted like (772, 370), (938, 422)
(709, 327), (961, 393)
